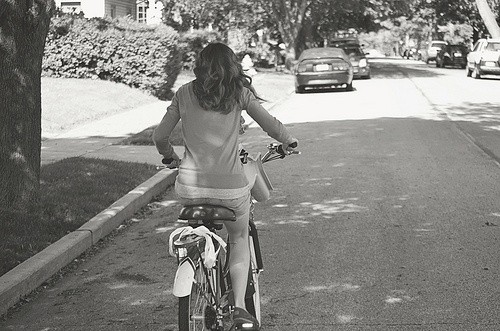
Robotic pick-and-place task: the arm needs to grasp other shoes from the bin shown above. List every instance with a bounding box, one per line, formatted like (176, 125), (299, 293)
(234, 307), (260, 327)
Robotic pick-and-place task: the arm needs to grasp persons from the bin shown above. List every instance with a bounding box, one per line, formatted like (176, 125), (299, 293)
(151, 41), (298, 330)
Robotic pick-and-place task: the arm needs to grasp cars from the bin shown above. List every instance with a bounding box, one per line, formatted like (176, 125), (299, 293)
(465, 38), (500, 79)
(290, 46), (355, 93)
(424, 40), (449, 64)
(435, 43), (470, 69)
(328, 36), (371, 79)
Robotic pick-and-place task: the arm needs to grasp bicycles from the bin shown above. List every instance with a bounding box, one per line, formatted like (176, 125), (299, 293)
(155, 142), (302, 331)
